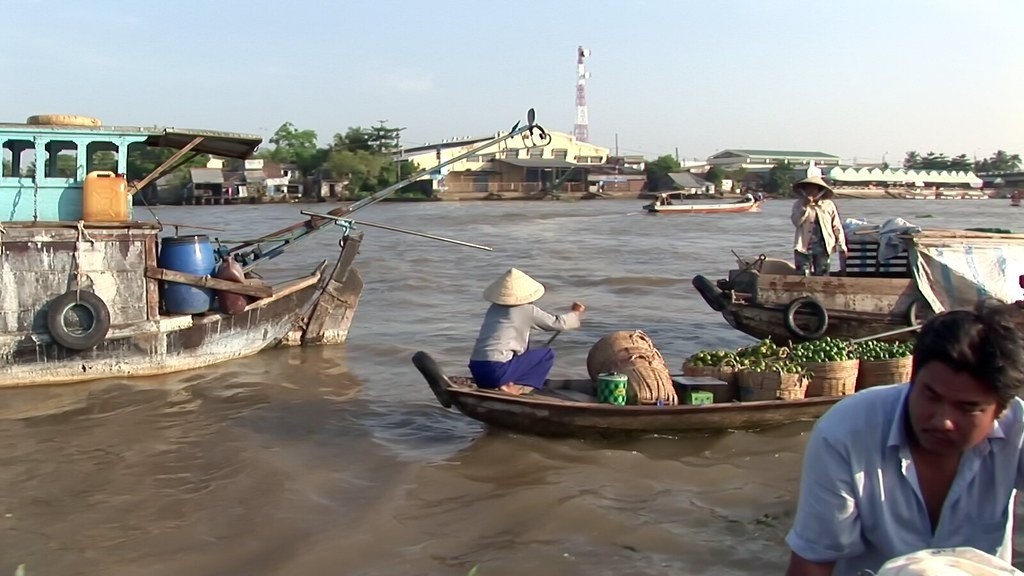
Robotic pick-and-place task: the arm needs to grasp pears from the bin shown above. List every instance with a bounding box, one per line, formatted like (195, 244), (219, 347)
(690, 336), (913, 373)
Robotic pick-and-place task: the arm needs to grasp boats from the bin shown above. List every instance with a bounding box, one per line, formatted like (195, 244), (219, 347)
(0, 107), (552, 390)
(411, 347), (856, 436)
(693, 217), (1024, 349)
(641, 190), (763, 213)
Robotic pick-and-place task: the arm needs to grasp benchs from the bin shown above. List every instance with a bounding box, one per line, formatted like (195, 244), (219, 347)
(671, 376), (728, 404)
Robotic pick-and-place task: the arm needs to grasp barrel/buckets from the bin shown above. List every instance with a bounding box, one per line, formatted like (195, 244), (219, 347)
(597, 371), (628, 405)
(158, 235), (215, 314)
(83, 171), (128, 229)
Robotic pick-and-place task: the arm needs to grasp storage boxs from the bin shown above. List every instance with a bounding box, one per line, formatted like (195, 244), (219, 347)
(680, 390), (714, 405)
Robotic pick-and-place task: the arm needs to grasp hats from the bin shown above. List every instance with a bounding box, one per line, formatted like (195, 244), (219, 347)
(484, 267), (545, 305)
(793, 175), (835, 197)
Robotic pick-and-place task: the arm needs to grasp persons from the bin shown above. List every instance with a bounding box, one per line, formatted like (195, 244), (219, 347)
(741, 189), (764, 202)
(791, 173), (848, 276)
(783, 296), (1024, 576)
(467, 267), (585, 395)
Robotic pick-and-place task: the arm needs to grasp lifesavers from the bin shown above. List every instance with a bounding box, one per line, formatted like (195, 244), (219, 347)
(411, 348), (454, 411)
(907, 300), (929, 335)
(691, 274), (728, 311)
(47, 289), (111, 351)
(785, 296), (829, 340)
